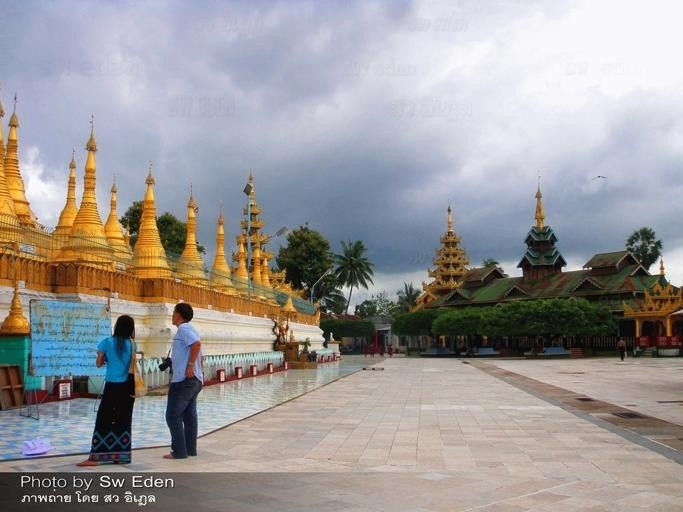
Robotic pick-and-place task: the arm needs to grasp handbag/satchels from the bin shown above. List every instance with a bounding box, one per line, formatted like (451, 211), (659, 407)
(128, 338), (147, 398)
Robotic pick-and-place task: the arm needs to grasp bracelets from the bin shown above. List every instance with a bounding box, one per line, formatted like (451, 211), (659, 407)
(188, 361), (193, 369)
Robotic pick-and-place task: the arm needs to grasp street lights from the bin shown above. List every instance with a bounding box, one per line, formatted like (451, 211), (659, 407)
(242, 183), (253, 298)
(310, 268), (332, 305)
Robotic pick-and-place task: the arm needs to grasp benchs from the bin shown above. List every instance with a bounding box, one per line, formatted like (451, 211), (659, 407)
(421, 347), (570, 358)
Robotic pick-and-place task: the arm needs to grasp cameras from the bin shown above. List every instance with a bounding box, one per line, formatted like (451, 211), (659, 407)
(157, 356), (172, 373)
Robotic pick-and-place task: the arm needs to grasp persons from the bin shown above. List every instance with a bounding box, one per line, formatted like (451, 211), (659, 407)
(616, 337), (626, 362)
(161, 302), (206, 460)
(341, 340), (393, 359)
(74, 313), (138, 468)
